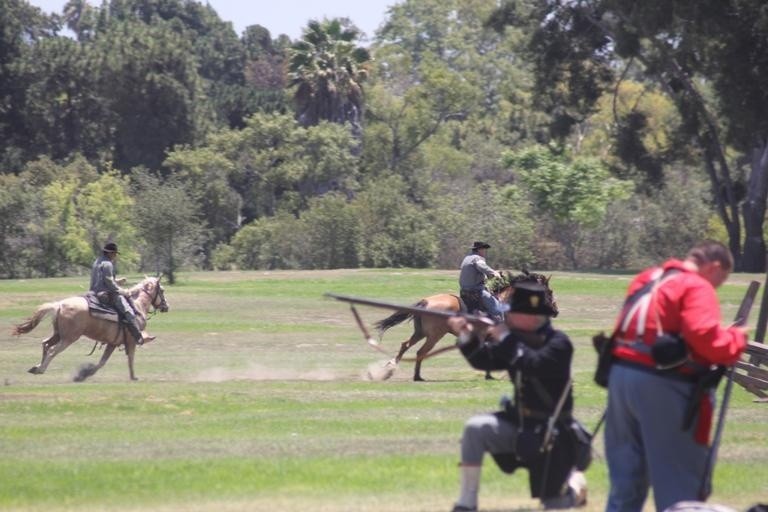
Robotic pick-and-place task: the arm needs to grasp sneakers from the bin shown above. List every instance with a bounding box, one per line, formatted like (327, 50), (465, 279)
(138, 336), (156, 346)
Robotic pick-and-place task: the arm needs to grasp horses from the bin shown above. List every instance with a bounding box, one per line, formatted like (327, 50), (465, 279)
(9, 272), (168, 381)
(373, 269), (558, 381)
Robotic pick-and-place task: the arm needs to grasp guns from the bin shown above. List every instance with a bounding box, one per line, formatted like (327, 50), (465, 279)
(324, 293), (496, 338)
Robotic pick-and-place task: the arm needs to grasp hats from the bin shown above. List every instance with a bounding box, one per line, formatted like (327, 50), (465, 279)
(500, 282), (558, 317)
(468, 242), (492, 249)
(102, 242), (121, 257)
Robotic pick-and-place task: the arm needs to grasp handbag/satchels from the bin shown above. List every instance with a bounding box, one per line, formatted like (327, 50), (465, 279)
(459, 288), (480, 309)
(562, 421), (591, 471)
(593, 333), (612, 387)
(96, 290), (112, 304)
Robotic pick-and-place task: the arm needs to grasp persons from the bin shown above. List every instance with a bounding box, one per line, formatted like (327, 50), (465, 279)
(446, 277), (586, 512)
(89, 242), (155, 344)
(459, 240), (502, 319)
(604, 238), (752, 512)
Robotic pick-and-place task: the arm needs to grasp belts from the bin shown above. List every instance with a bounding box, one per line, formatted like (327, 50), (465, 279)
(513, 399), (573, 423)
